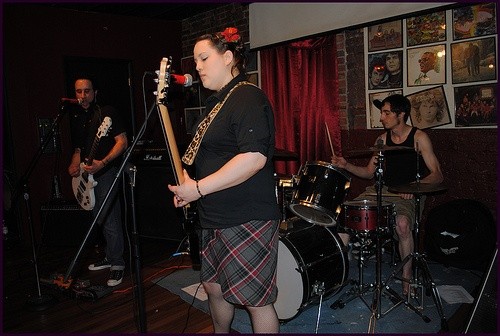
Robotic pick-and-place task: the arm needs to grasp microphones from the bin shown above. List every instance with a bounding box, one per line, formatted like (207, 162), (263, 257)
(169, 73), (193, 88)
(61, 97), (82, 105)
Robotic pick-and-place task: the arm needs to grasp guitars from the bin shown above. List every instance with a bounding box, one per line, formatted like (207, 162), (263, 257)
(71, 116), (113, 211)
(152, 56), (203, 272)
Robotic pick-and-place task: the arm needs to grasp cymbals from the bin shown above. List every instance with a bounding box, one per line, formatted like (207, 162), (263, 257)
(274, 148), (298, 162)
(344, 144), (415, 156)
(389, 182), (452, 193)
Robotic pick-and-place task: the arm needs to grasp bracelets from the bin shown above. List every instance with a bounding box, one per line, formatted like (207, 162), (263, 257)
(196, 180), (204, 197)
(102, 160), (106, 166)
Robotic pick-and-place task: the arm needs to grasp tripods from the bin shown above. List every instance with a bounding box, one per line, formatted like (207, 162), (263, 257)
(328, 153), (450, 331)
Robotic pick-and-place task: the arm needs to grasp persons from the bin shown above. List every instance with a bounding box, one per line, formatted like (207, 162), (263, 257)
(68, 73), (127, 287)
(168, 28), (282, 333)
(330, 94), (443, 297)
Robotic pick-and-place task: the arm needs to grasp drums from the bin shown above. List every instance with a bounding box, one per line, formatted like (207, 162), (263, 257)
(343, 199), (393, 233)
(271, 216), (350, 323)
(288, 160), (352, 226)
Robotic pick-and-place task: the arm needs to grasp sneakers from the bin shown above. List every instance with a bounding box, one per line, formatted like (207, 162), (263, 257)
(107, 270), (124, 286)
(89, 259), (112, 270)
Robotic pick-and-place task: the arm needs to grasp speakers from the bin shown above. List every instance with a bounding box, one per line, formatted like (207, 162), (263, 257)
(40, 202), (104, 260)
(128, 148), (190, 241)
